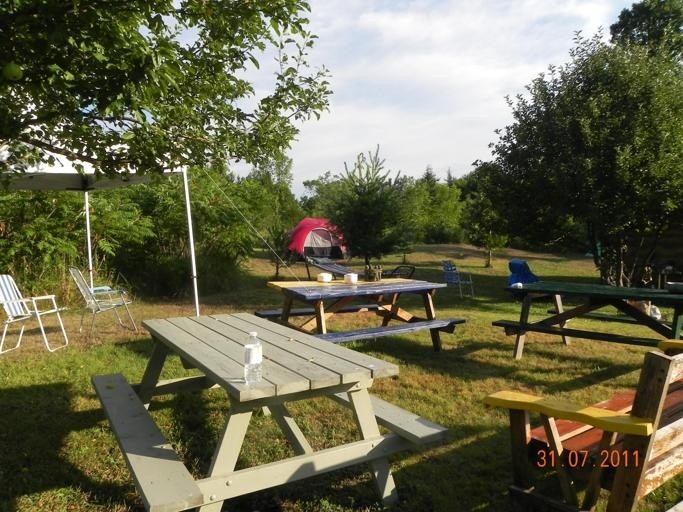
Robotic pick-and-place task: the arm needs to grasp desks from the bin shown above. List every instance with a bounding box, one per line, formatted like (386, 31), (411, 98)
(510, 283), (683, 362)
(137, 312), (400, 511)
(266, 278), (448, 358)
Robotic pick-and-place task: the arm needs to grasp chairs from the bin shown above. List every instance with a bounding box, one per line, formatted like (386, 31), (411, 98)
(391, 264), (416, 278)
(67, 265), (138, 338)
(484, 340), (683, 511)
(509, 259), (558, 301)
(0, 274), (69, 354)
(304, 252), (390, 280)
(441, 260), (475, 299)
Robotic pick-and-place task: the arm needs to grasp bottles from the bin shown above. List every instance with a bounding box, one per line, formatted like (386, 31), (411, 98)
(244, 331), (264, 384)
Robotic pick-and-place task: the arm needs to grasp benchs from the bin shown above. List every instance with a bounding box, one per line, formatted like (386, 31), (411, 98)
(311, 317), (466, 343)
(330, 388), (451, 448)
(492, 319), (666, 362)
(91, 373), (205, 512)
(548, 307), (672, 331)
(254, 303), (381, 317)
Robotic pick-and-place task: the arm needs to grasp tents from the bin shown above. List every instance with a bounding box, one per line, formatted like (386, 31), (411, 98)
(280, 216), (346, 262)
